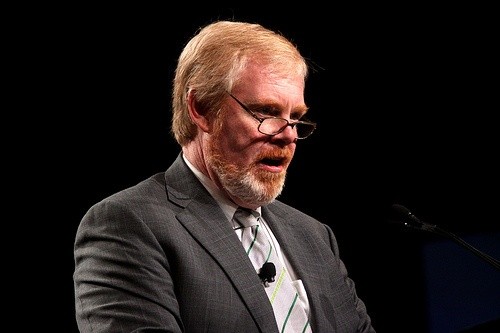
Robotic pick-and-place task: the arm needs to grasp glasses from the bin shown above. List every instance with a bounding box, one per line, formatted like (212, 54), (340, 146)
(218, 87), (317, 139)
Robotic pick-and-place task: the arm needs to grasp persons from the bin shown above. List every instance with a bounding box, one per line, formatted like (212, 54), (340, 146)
(72, 21), (376, 333)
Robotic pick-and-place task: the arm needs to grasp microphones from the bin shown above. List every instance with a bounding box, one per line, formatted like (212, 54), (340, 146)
(260, 262), (276, 282)
(391, 204), (500, 268)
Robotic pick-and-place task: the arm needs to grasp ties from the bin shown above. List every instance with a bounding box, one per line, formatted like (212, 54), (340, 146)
(233, 209), (312, 333)
